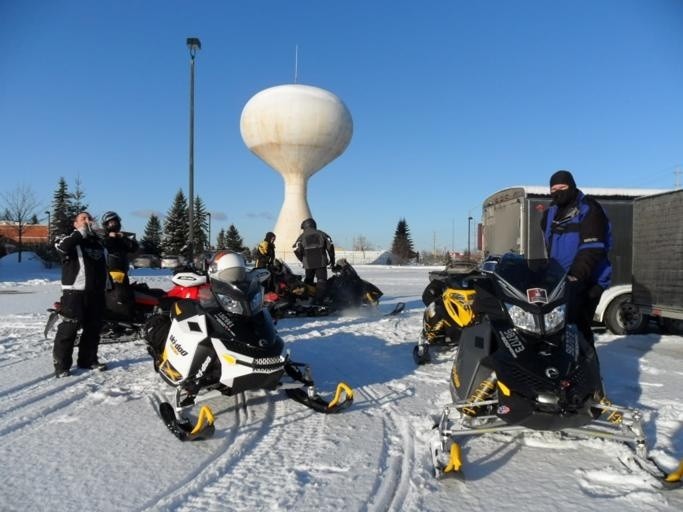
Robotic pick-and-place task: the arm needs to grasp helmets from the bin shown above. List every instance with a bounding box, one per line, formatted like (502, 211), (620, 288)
(301, 218), (316, 231)
(100, 211), (120, 233)
(208, 250), (246, 283)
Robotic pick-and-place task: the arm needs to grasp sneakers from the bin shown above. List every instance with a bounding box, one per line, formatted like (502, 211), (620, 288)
(77, 361), (106, 371)
(55, 367), (71, 378)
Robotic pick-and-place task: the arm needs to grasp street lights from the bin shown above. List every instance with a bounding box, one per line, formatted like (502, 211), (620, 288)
(207, 211), (212, 246)
(186, 36), (201, 248)
(468, 215), (475, 254)
(44, 211), (52, 244)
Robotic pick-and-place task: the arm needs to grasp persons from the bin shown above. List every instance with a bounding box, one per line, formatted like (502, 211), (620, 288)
(47, 210), (111, 376)
(537, 171), (615, 408)
(98, 211), (139, 337)
(253, 232), (276, 268)
(292, 216), (335, 282)
